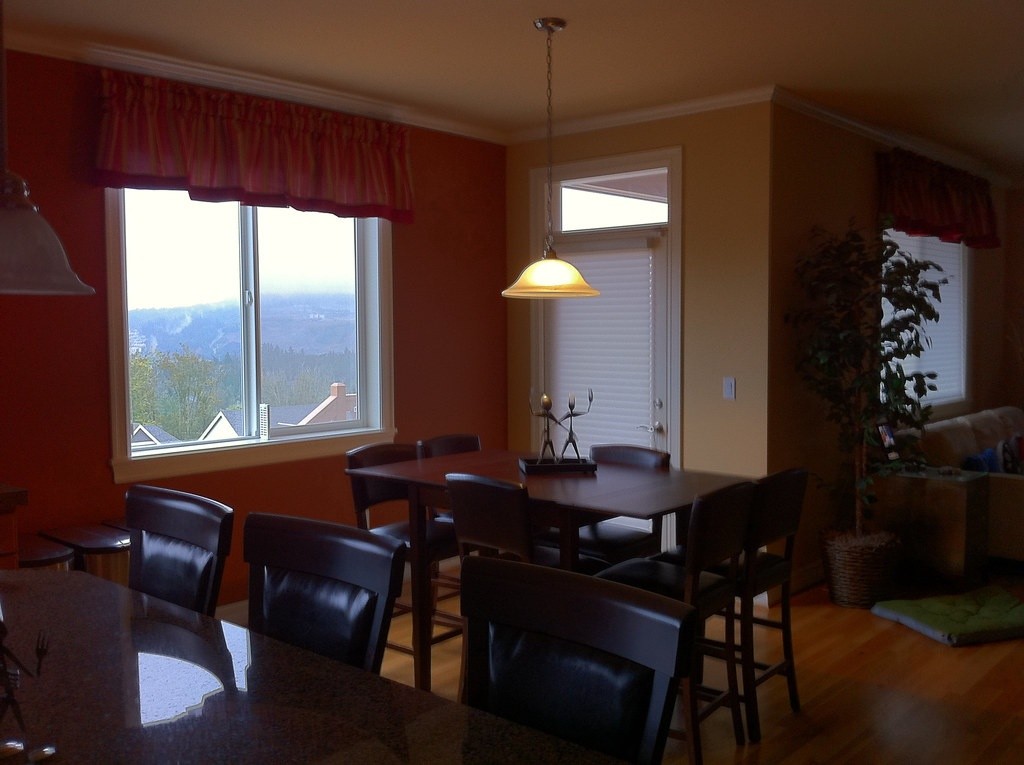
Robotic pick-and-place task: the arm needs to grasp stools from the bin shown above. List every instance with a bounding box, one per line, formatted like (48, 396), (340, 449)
(20, 516), (132, 572)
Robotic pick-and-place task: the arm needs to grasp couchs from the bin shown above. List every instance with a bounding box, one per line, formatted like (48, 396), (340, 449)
(889, 407), (1023, 561)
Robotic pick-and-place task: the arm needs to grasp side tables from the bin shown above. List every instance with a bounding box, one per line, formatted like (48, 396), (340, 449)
(864, 461), (989, 581)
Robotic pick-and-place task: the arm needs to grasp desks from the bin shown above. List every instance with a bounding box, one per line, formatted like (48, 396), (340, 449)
(0, 565), (608, 765)
(344, 451), (758, 695)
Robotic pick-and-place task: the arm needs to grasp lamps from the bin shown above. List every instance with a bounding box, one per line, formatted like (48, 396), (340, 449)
(498, 16), (600, 298)
(0, 171), (97, 295)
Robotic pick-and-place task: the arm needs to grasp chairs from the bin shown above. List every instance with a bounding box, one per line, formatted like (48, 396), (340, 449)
(542, 446), (672, 568)
(236, 510), (414, 677)
(344, 441), (500, 692)
(651, 467), (805, 744)
(123, 485), (236, 619)
(416, 434), (481, 531)
(458, 556), (702, 765)
(444, 472), (586, 705)
(591, 481), (756, 764)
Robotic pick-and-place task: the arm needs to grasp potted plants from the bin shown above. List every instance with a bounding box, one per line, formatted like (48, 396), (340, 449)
(780, 213), (951, 608)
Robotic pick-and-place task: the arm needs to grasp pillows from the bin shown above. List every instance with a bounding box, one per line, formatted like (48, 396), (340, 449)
(962, 433), (1023, 475)
